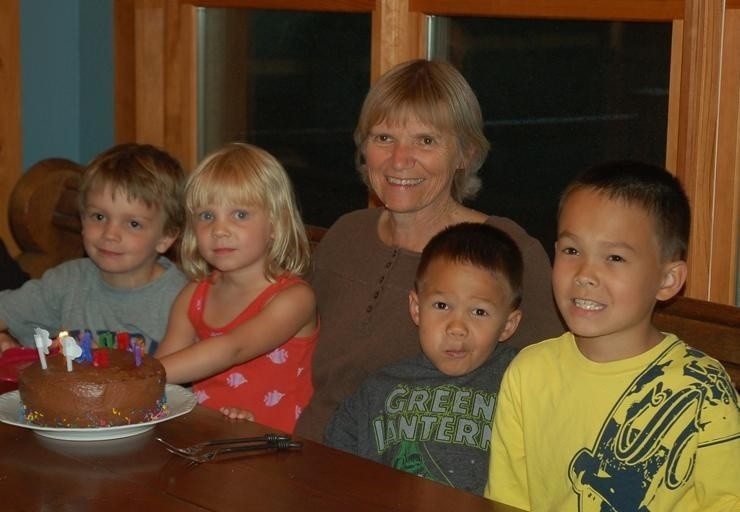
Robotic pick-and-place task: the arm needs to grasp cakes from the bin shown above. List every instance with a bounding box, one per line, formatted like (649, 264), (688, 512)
(16, 346), (168, 428)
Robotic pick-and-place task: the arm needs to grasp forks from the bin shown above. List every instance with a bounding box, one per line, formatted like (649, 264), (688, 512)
(154, 433), (289, 458)
(164, 439), (304, 464)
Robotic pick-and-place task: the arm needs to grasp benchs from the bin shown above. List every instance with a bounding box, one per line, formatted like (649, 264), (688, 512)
(9, 156), (740, 394)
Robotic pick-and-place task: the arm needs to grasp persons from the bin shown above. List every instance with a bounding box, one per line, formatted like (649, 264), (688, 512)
(320, 223), (530, 497)
(153, 140), (320, 433)
(0, 141), (187, 361)
(478, 158), (738, 509)
(294, 58), (565, 450)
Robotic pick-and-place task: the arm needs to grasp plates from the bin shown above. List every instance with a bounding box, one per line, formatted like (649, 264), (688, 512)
(0, 382), (198, 441)
